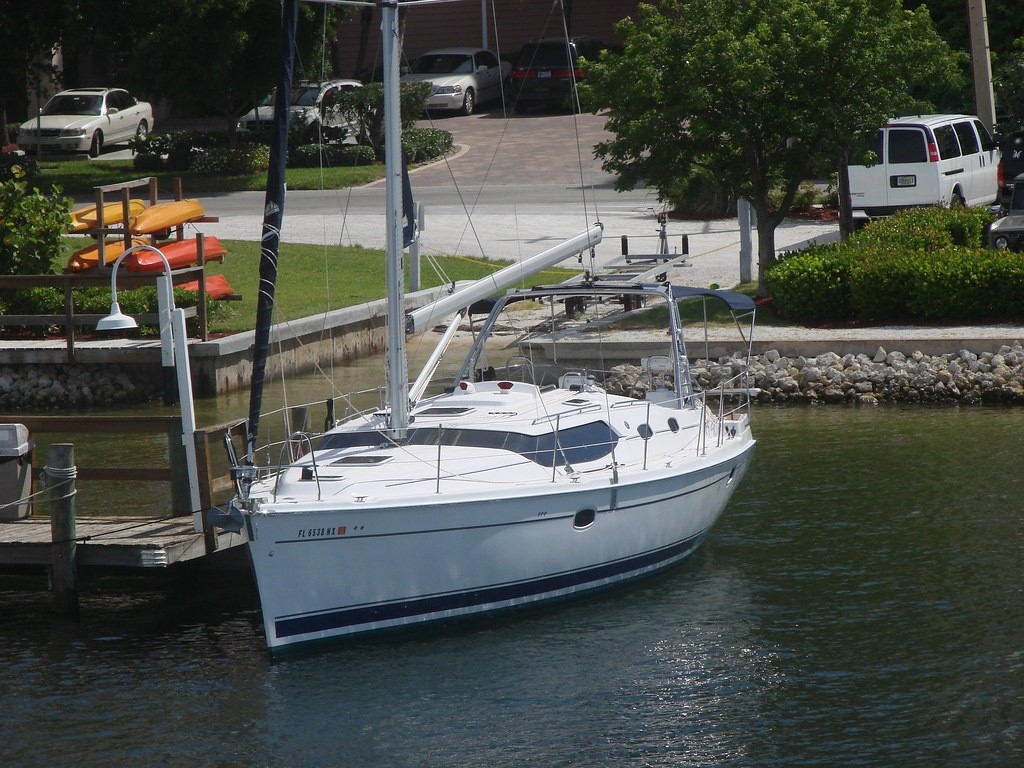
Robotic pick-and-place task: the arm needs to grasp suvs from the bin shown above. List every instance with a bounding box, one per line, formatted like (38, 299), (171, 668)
(507, 33), (609, 114)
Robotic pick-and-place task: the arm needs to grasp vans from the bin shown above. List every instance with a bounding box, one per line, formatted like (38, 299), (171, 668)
(835, 112), (1003, 232)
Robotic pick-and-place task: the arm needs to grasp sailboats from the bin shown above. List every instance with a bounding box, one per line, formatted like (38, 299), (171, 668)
(205, 1), (758, 664)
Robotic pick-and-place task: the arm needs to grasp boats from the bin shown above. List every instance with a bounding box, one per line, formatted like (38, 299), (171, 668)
(171, 274), (236, 302)
(66, 198), (148, 234)
(127, 198), (207, 236)
(66, 236), (151, 274)
(126, 235), (227, 275)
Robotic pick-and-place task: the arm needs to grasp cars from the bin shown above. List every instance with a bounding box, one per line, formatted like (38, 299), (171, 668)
(987, 129), (1024, 253)
(398, 46), (513, 117)
(16, 87), (155, 157)
(234, 76), (378, 145)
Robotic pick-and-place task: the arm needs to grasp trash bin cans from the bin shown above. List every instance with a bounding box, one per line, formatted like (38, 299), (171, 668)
(0, 422), (35, 520)
